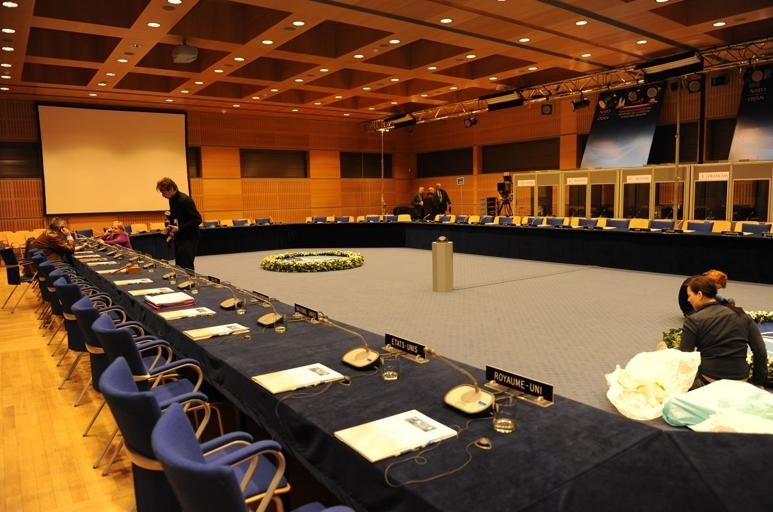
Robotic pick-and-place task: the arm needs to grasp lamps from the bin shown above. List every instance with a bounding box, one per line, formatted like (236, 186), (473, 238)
(463, 116), (479, 128)
(541, 104), (554, 115)
(570, 99), (590, 112)
(647, 84), (665, 99)
(628, 88), (643, 102)
(687, 79), (702, 94)
(599, 96), (618, 109)
(748, 67), (763, 83)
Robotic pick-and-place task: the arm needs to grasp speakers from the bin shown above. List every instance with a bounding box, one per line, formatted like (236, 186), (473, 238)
(671, 82), (678, 91)
(711, 76), (727, 86)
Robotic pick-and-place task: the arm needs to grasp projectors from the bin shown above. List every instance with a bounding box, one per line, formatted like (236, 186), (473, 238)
(171, 46), (199, 64)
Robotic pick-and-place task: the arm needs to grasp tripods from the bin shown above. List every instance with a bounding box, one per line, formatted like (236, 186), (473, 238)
(498, 195), (513, 216)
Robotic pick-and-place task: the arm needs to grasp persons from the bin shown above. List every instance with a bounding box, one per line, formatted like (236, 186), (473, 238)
(423, 187), (439, 219)
(676, 275), (768, 392)
(155, 176), (201, 274)
(96, 221), (132, 249)
(27, 216), (75, 273)
(678, 269), (734, 317)
(433, 183), (451, 215)
(411, 186), (425, 219)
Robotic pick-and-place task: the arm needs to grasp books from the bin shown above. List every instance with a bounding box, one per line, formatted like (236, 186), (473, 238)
(183, 323), (250, 341)
(113, 278), (153, 286)
(252, 364), (345, 394)
(128, 287), (195, 311)
(334, 408), (457, 462)
(157, 306), (216, 322)
(74, 250), (126, 275)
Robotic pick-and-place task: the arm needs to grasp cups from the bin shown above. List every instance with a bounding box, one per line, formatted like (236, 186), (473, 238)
(189, 281), (199, 295)
(232, 298), (247, 317)
(493, 395), (516, 437)
(456, 219), (459, 223)
(168, 274), (177, 286)
(271, 313), (287, 334)
(147, 263), (156, 272)
(378, 355), (400, 382)
(415, 220), (419, 223)
(522, 221), (529, 228)
(502, 222), (507, 226)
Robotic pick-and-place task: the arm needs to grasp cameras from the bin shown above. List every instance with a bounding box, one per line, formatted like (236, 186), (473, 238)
(164, 211), (178, 244)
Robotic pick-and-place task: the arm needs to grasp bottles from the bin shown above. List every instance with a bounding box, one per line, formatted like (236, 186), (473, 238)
(314, 218), (317, 224)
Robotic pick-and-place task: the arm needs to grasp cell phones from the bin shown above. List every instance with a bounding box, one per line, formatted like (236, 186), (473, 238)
(61, 226), (64, 231)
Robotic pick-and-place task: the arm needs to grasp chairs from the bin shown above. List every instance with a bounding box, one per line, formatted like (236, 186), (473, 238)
(0, 211), (772, 510)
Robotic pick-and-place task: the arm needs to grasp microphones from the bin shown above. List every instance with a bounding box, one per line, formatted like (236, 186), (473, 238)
(423, 346), (495, 415)
(69, 228), (282, 327)
(318, 317), (379, 370)
